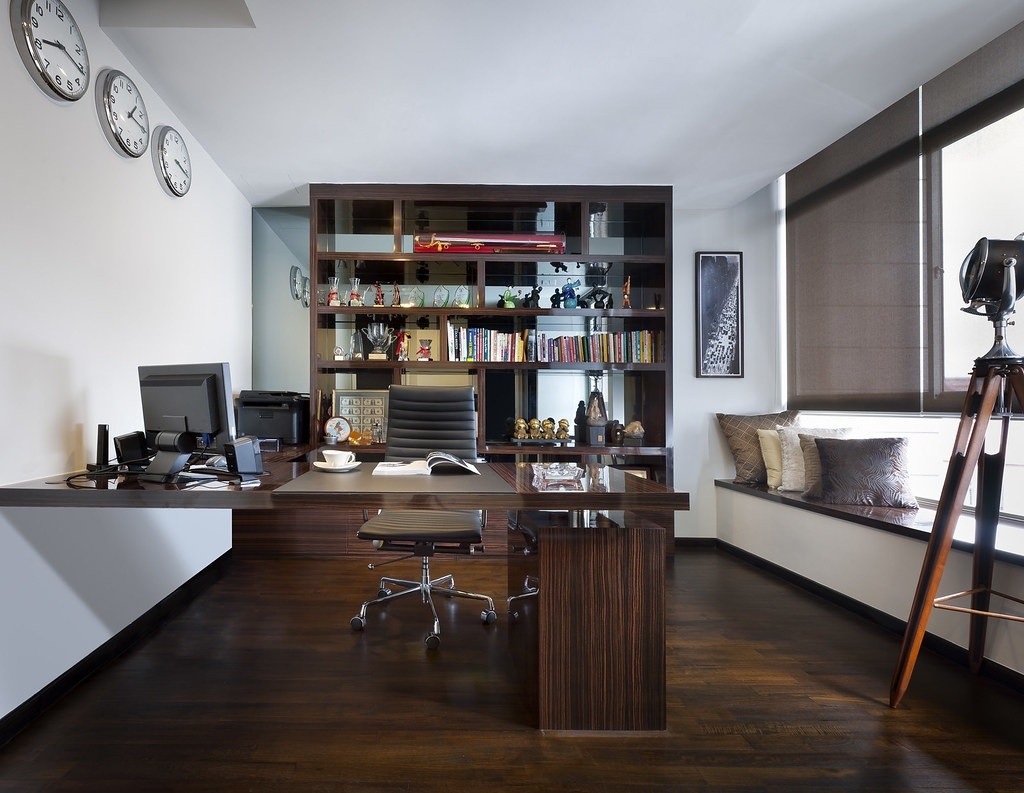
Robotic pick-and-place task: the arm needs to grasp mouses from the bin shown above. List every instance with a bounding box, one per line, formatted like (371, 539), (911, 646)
(205, 455), (227, 467)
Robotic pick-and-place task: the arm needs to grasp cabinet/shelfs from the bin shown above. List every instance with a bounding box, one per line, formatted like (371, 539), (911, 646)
(308, 182), (674, 482)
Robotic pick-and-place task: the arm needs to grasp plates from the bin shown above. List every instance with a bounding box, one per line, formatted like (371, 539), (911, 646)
(324, 416), (352, 442)
(312, 461), (362, 473)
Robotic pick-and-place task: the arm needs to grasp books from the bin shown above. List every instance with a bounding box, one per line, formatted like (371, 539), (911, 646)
(371, 451), (483, 477)
(446, 320), (666, 363)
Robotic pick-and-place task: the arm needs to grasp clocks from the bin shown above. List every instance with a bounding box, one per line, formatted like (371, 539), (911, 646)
(10, 0), (90, 102)
(95, 68), (150, 159)
(301, 277), (311, 308)
(290, 266), (303, 300)
(151, 126), (192, 198)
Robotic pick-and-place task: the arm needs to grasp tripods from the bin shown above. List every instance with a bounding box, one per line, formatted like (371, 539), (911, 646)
(888, 359), (1024, 707)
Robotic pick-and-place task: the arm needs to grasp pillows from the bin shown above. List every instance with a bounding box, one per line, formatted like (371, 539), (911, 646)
(757, 429), (783, 491)
(716, 410), (801, 483)
(775, 424), (853, 492)
(799, 432), (824, 500)
(815, 437), (920, 508)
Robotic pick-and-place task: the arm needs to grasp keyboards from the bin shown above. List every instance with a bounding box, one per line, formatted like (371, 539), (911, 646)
(190, 466), (272, 477)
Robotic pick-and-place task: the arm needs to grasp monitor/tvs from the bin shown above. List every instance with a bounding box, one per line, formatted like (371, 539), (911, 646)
(138, 363), (237, 485)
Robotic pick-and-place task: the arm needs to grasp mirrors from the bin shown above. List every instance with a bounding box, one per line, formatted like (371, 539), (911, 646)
(251, 205), (310, 396)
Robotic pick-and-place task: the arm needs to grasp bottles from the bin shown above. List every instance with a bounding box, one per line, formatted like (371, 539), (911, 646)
(349, 328), (365, 360)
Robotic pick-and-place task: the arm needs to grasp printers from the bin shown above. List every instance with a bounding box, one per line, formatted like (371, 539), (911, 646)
(234, 390), (310, 446)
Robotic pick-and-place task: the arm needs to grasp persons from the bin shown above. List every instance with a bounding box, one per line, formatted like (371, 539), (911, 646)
(502, 275), (633, 308)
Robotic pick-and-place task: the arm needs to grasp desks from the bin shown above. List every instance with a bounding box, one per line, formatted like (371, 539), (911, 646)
(1, 450), (690, 732)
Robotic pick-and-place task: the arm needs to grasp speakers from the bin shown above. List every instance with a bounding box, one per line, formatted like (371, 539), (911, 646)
(223, 435), (263, 484)
(115, 431), (151, 471)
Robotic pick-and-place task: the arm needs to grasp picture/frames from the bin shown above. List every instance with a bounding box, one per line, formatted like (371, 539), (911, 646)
(332, 389), (389, 443)
(695, 251), (745, 377)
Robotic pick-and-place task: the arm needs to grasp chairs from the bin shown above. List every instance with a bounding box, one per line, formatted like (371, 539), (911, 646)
(349, 385), (497, 650)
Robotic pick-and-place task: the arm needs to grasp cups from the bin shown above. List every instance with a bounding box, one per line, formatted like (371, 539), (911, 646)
(322, 449), (355, 467)
(349, 278), (360, 300)
(327, 277), (340, 300)
